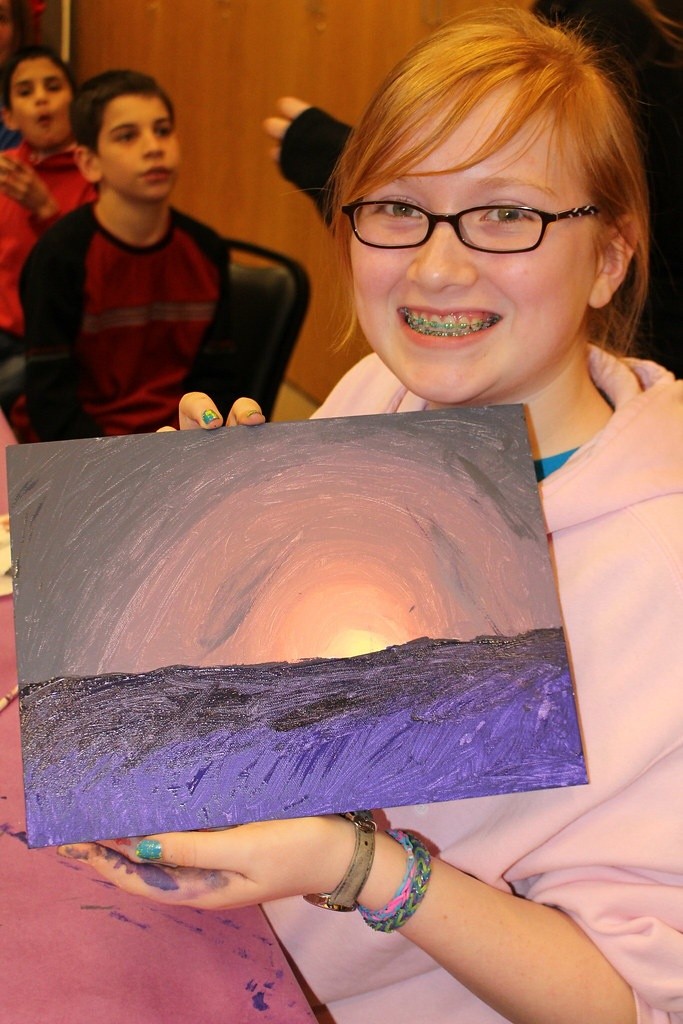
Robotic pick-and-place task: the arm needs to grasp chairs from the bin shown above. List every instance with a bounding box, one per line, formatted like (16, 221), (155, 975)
(212, 242), (310, 421)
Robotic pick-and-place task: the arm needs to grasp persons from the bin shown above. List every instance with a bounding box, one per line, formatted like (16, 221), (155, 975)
(17, 66), (259, 435)
(261, 0), (683, 378)
(1, 0), (45, 152)
(0, 41), (101, 413)
(47, 12), (683, 1024)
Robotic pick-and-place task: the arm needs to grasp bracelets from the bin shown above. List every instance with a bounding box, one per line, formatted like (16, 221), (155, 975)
(356, 829), (415, 921)
(301, 808), (378, 913)
(362, 833), (430, 933)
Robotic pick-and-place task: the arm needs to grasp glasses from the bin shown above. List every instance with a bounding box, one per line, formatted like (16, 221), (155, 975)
(341, 201), (599, 254)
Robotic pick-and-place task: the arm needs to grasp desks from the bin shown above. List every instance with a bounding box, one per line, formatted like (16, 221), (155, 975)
(0, 409), (320, 1024)
